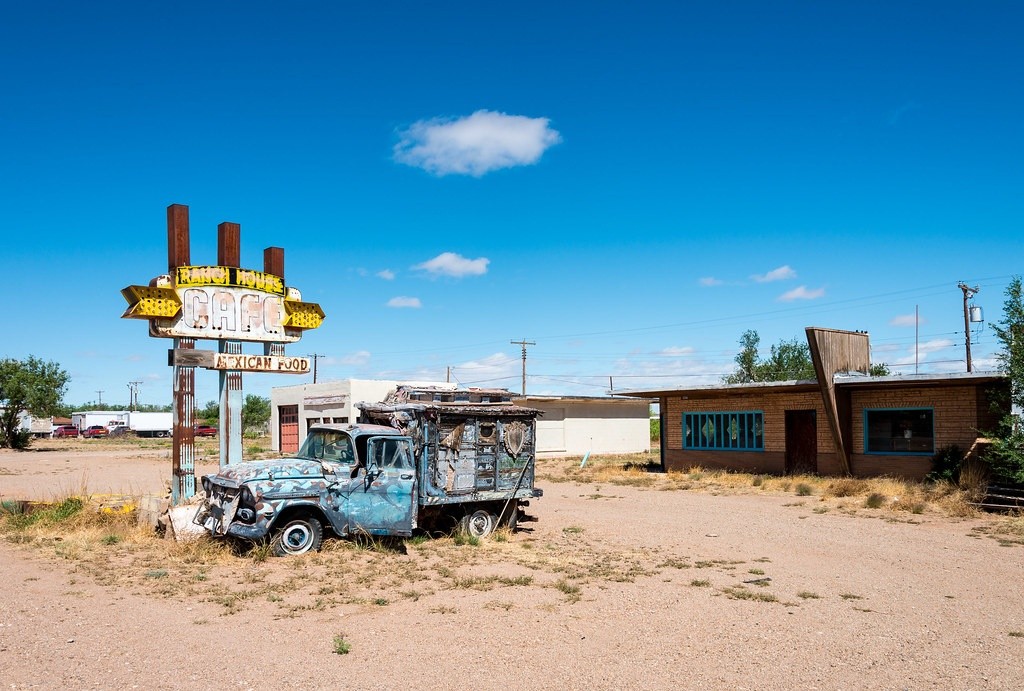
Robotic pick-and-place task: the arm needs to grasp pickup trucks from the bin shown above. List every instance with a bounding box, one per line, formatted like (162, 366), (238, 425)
(194, 425), (217, 438)
(82, 425), (107, 439)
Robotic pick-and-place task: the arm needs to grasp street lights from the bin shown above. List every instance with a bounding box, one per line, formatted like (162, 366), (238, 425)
(126, 385), (133, 413)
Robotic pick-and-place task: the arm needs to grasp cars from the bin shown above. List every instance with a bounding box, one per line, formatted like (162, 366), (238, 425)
(55, 425), (79, 438)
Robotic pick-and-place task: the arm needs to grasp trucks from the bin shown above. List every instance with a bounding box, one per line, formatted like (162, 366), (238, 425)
(192, 397), (546, 561)
(21, 414), (52, 439)
(105, 411), (174, 439)
